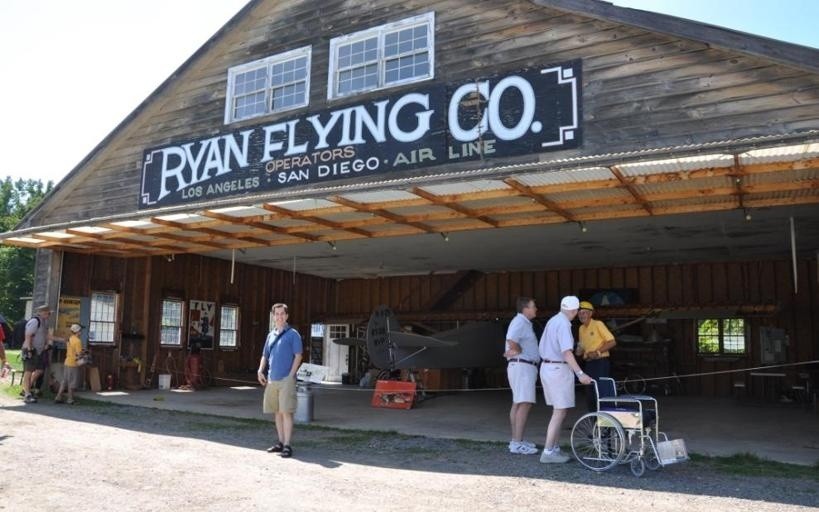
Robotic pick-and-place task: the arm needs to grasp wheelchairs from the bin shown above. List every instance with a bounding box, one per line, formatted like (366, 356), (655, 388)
(570, 376), (690, 477)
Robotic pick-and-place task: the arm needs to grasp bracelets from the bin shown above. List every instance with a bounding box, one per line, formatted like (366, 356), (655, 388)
(596, 351), (600, 357)
(576, 370), (583, 377)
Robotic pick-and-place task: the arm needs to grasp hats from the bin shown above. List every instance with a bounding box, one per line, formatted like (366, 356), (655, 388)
(70, 322), (86, 334)
(560, 296), (579, 310)
(578, 301), (594, 311)
(37, 305), (49, 312)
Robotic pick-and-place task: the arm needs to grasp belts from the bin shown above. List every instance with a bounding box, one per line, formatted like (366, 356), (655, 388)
(542, 360), (568, 364)
(508, 359), (537, 366)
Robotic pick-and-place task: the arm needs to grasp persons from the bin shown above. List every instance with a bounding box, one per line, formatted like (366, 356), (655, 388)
(54, 323), (85, 405)
(0, 324), (11, 377)
(503, 297), (541, 455)
(19, 305), (50, 402)
(539, 295), (591, 464)
(257, 303), (304, 457)
(576, 301), (617, 439)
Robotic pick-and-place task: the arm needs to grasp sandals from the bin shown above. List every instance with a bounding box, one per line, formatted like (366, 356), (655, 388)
(267, 442), (283, 452)
(281, 445), (293, 458)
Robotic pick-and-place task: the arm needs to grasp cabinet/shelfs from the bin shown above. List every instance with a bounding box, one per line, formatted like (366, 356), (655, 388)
(400, 367), (441, 388)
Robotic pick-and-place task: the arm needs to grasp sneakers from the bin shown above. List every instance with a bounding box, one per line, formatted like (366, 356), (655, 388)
(54, 395), (64, 403)
(24, 393), (37, 403)
(510, 442), (539, 455)
(540, 450), (571, 464)
(553, 446), (576, 460)
(67, 398), (80, 405)
(20, 389), (34, 397)
(509, 440), (537, 448)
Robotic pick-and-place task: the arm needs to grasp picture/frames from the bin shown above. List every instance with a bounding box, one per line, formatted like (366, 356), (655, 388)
(187, 300), (216, 350)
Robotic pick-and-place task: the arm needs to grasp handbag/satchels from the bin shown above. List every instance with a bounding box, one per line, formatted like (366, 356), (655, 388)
(75, 349), (88, 367)
(262, 360), (270, 380)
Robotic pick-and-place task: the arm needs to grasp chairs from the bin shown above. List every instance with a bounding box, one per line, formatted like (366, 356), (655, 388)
(11, 353), (24, 385)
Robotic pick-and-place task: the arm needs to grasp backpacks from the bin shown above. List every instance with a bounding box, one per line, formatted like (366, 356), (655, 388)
(8, 316), (41, 350)
